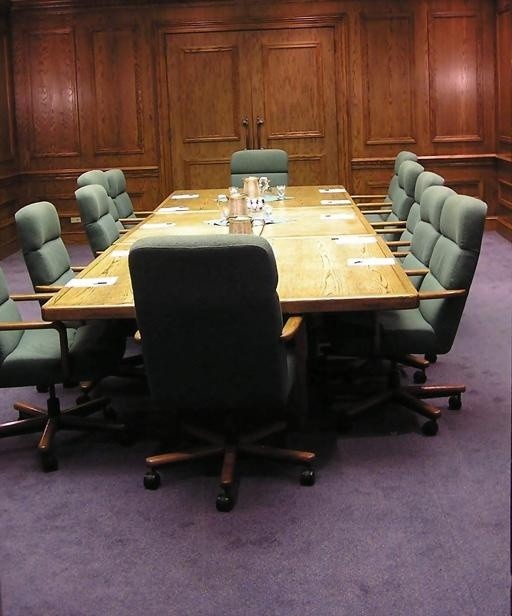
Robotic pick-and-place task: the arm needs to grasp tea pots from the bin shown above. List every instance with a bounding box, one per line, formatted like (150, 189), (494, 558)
(215, 192), (252, 218)
(224, 213), (266, 238)
(240, 176), (265, 201)
